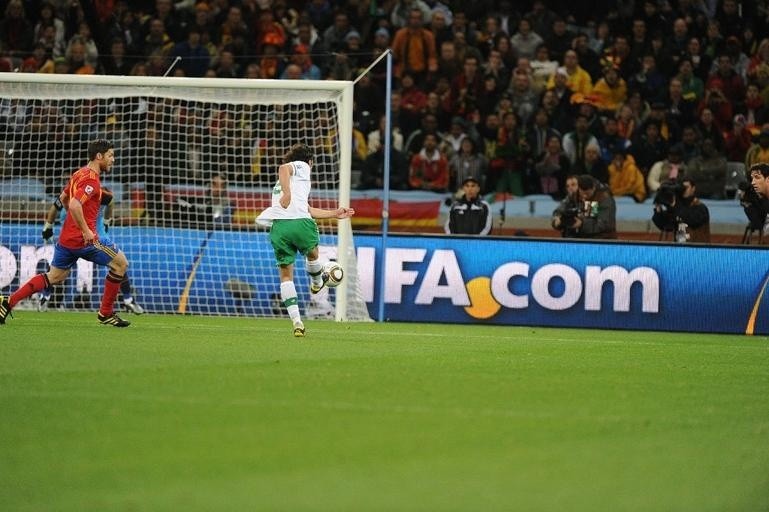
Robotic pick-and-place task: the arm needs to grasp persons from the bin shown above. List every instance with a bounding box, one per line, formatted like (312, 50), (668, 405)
(0, 140), (130, 326)
(254, 144), (356, 337)
(443, 175), (493, 235)
(652, 177), (710, 243)
(741, 162), (769, 245)
(1, 2), (768, 200)
(203, 173), (237, 230)
(551, 175), (617, 239)
(96, 188), (144, 315)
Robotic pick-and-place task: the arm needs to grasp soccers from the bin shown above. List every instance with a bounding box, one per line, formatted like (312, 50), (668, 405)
(323, 261), (343, 287)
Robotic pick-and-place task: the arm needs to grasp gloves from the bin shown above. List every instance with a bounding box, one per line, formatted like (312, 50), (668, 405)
(103, 218), (114, 235)
(41, 221), (55, 245)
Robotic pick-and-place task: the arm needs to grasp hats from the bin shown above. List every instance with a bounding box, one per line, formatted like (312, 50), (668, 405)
(462, 175), (481, 185)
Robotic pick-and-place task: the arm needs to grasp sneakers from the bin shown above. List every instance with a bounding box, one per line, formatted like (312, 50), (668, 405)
(293, 328), (307, 338)
(97, 310), (131, 328)
(0, 295), (10, 324)
(35, 291), (51, 312)
(123, 297), (144, 316)
(311, 271), (330, 295)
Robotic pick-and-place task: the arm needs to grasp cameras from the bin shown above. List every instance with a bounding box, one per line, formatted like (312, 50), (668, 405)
(711, 92), (719, 97)
